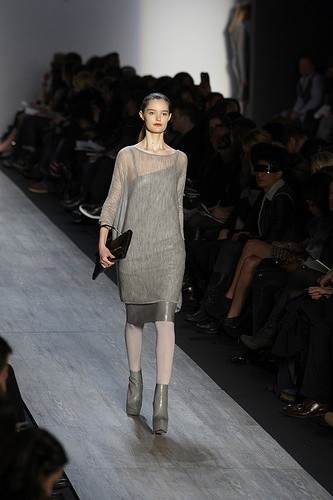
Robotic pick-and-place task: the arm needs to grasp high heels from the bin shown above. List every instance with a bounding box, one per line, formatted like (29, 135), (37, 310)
(241, 322), (277, 355)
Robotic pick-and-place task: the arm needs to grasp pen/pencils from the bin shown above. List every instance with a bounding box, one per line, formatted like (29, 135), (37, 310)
(217, 200), (221, 207)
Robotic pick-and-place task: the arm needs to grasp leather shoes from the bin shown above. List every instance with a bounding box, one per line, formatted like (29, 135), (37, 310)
(194, 317), (242, 336)
(280, 398), (333, 418)
(184, 310), (221, 323)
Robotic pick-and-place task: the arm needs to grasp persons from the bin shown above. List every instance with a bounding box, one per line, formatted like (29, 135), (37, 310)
(228, 0), (252, 112)
(0, 334), (70, 500)
(0, 51), (333, 427)
(99, 93), (188, 435)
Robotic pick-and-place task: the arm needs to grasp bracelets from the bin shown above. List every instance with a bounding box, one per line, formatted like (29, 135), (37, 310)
(286, 241), (292, 250)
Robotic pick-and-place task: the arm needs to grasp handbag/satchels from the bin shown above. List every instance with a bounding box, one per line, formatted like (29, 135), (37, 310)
(92, 225), (133, 280)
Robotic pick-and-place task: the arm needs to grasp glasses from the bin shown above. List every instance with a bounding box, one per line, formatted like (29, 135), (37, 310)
(254, 164), (277, 173)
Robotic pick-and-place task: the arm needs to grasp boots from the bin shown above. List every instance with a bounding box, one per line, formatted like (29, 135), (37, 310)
(153, 383), (168, 435)
(126, 369), (143, 415)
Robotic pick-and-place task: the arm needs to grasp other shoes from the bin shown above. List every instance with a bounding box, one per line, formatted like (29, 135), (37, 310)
(20, 168), (43, 181)
(0, 140), (14, 152)
(79, 203), (104, 219)
(28, 184), (49, 193)
